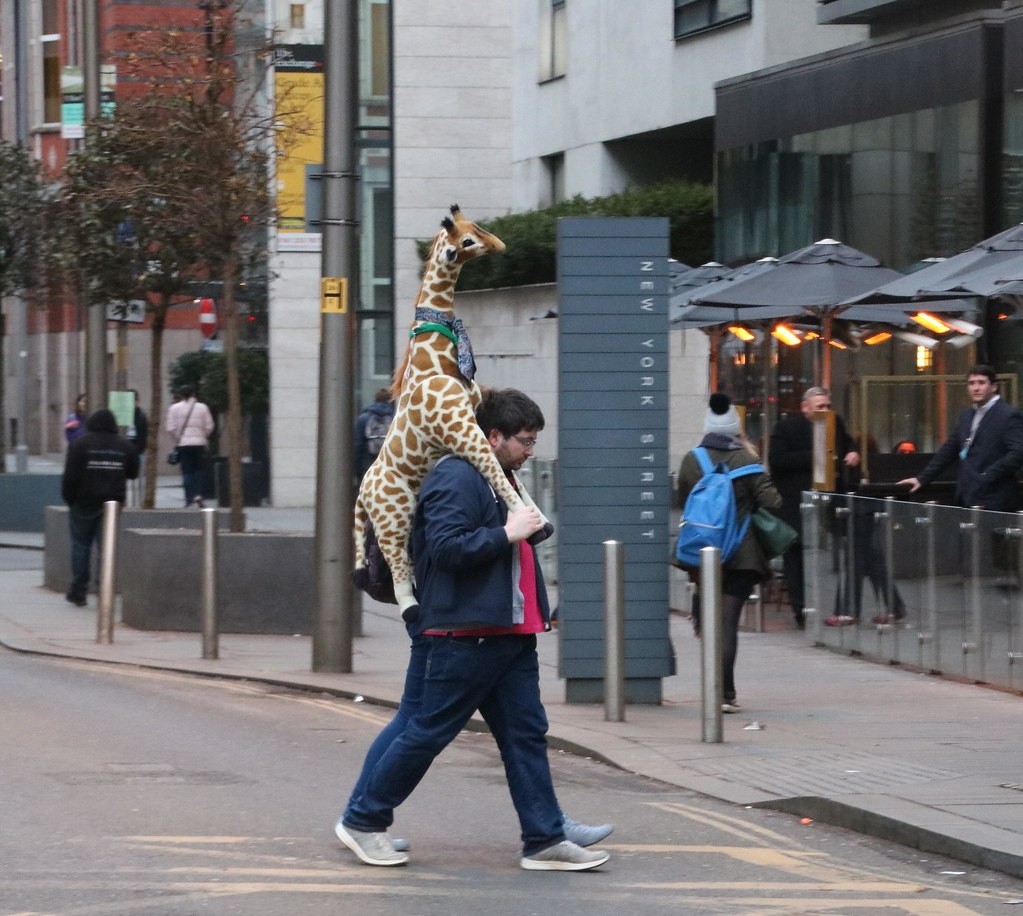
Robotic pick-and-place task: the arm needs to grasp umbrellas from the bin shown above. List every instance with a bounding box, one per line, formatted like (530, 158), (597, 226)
(833, 212), (1023, 444)
(666, 232), (907, 394)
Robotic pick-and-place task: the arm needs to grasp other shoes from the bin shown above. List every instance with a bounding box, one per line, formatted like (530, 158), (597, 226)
(825, 614), (863, 625)
(65, 590), (87, 606)
(871, 606), (908, 624)
(721, 697), (740, 713)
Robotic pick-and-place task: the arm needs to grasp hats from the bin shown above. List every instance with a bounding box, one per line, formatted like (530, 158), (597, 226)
(703, 392), (740, 436)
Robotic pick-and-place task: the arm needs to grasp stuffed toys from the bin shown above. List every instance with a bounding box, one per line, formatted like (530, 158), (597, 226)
(351, 205), (555, 621)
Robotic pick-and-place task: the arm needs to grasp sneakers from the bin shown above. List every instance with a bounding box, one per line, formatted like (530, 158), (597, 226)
(562, 813), (615, 848)
(390, 838), (410, 851)
(521, 840), (610, 871)
(335, 822), (410, 866)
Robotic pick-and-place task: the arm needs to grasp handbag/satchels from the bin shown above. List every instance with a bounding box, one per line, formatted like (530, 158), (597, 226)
(168, 451), (180, 465)
(751, 506), (799, 559)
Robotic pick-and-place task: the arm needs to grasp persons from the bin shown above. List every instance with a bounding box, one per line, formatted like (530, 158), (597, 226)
(351, 387), (396, 480)
(334, 390), (618, 870)
(167, 385), (216, 507)
(824, 432), (907, 623)
(127, 389), (149, 456)
(65, 411), (140, 605)
(678, 392), (783, 712)
(894, 366), (1023, 589)
(769, 388), (862, 630)
(66, 393), (91, 440)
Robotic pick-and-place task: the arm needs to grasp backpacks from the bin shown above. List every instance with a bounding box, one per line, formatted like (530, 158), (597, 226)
(675, 447), (768, 568)
(357, 519), (416, 605)
(365, 410), (396, 455)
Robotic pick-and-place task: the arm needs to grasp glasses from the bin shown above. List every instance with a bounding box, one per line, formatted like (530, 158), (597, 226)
(510, 431), (537, 450)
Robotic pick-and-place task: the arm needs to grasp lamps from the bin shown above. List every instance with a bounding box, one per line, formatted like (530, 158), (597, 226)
(770, 326), (801, 346)
(945, 317), (983, 338)
(892, 330), (941, 352)
(946, 333), (977, 351)
(863, 331), (890, 346)
(905, 310), (950, 334)
(728, 325), (755, 342)
